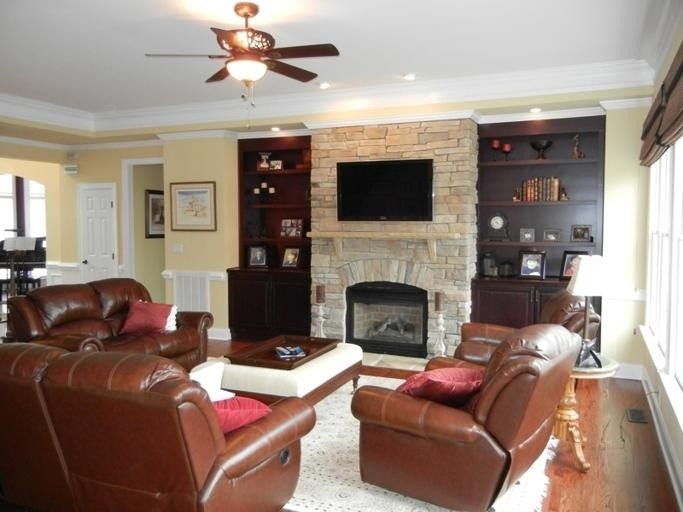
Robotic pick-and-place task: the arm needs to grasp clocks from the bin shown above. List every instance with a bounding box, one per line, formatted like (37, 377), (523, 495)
(485, 211), (513, 242)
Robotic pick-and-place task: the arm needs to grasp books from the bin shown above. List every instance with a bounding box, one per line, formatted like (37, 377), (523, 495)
(274, 345), (307, 359)
(513, 174), (560, 203)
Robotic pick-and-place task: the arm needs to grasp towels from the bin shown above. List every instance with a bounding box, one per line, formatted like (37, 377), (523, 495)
(3, 237), (36, 252)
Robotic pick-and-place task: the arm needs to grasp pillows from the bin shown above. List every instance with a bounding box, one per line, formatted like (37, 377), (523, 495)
(121, 300), (175, 336)
(210, 391), (275, 436)
(395, 367), (486, 404)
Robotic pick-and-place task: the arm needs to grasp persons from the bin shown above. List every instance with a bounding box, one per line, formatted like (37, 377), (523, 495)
(566, 256), (580, 276)
(284, 251), (297, 266)
(521, 255), (543, 276)
(251, 249), (266, 264)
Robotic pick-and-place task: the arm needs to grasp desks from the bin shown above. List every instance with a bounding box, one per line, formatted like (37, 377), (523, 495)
(552, 352), (620, 474)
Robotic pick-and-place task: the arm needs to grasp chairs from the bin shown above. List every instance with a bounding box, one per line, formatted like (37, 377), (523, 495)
(351, 323), (582, 511)
(454, 286), (599, 391)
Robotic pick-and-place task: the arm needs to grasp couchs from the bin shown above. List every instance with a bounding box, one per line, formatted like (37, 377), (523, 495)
(3, 277), (217, 373)
(3, 343), (314, 512)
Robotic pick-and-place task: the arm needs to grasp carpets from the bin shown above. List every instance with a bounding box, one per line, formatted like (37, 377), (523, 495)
(187, 357), (557, 511)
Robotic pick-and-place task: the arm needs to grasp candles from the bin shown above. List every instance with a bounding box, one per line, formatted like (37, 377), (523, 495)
(268, 187), (275, 194)
(253, 188), (260, 194)
(502, 144), (512, 153)
(492, 138), (500, 148)
(260, 182), (268, 188)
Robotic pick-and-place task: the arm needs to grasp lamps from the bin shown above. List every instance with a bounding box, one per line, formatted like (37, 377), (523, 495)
(225, 58), (268, 133)
(565, 256), (604, 367)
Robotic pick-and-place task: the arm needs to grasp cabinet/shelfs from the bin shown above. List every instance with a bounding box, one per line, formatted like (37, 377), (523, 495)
(238, 134), (311, 269)
(477, 114), (605, 279)
(471, 281), (601, 353)
(226, 266), (310, 340)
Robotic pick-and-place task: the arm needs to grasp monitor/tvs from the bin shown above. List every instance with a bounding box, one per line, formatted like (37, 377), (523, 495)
(336, 158), (433, 221)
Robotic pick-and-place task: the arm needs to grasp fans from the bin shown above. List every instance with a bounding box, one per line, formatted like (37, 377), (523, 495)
(144, 2), (339, 134)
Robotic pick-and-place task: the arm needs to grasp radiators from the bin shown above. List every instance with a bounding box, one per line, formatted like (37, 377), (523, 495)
(161, 271), (228, 312)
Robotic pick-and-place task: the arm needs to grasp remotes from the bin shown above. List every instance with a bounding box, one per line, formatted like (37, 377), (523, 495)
(285, 346), (297, 355)
(275, 347), (290, 355)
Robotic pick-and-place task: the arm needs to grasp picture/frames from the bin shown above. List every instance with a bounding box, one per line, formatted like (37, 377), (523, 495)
(280, 245), (302, 269)
(145, 189), (164, 238)
(519, 224), (592, 280)
(169, 181), (217, 231)
(247, 242), (267, 267)
